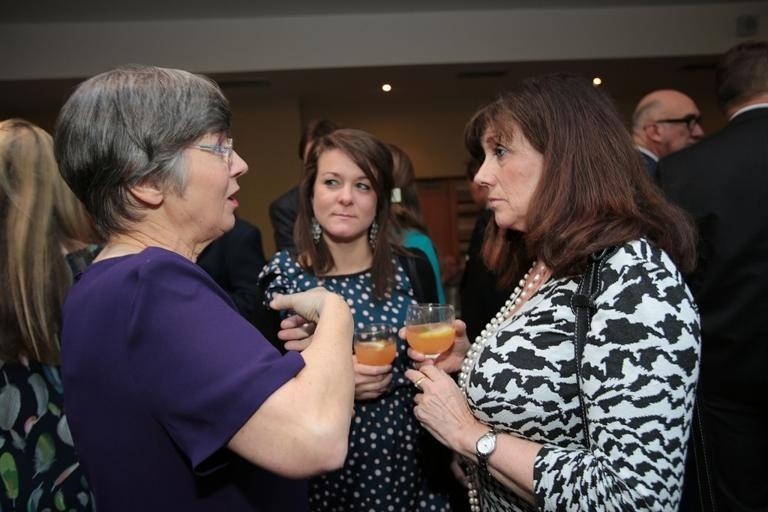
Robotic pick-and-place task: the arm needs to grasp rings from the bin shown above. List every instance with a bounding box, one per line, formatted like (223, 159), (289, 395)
(412, 376), (426, 390)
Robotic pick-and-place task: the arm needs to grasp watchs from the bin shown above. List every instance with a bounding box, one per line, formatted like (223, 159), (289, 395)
(475, 428), (508, 475)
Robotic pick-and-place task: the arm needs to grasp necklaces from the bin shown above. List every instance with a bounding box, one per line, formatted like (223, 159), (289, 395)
(458, 255), (546, 386)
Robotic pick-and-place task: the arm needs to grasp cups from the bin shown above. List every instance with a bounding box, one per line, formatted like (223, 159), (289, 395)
(351, 322), (398, 368)
(404, 300), (454, 366)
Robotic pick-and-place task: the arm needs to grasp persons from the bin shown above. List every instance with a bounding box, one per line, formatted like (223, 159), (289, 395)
(55, 63), (355, 511)
(632, 90), (703, 186)
(404, 74), (702, 511)
(269, 120), (357, 253)
(256, 129), (470, 511)
(0, 120), (106, 511)
(385, 144), (445, 322)
(461, 158), (538, 346)
(654, 41), (767, 512)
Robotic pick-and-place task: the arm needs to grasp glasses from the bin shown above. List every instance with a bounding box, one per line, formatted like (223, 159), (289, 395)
(190, 138), (234, 166)
(642, 114), (707, 130)
